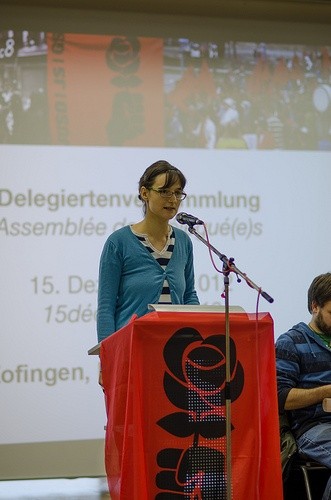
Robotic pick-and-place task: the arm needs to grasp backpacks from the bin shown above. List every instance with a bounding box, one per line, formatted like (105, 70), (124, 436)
(280, 423), (297, 483)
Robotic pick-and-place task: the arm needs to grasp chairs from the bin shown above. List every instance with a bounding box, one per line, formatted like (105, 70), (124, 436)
(289, 454), (328, 500)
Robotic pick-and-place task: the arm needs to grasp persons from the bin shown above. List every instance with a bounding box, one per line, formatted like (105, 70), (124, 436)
(95, 159), (202, 386)
(0, 51), (331, 153)
(274, 272), (331, 500)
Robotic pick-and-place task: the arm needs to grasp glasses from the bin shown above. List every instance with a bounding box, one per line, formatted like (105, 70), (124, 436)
(149, 187), (187, 200)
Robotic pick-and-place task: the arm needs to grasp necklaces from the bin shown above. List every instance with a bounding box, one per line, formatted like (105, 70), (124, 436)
(309, 322), (331, 347)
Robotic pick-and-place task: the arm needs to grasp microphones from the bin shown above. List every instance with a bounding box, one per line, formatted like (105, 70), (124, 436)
(176, 212), (203, 225)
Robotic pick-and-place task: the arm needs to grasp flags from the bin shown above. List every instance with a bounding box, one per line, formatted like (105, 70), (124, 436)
(168, 48), (307, 109)
